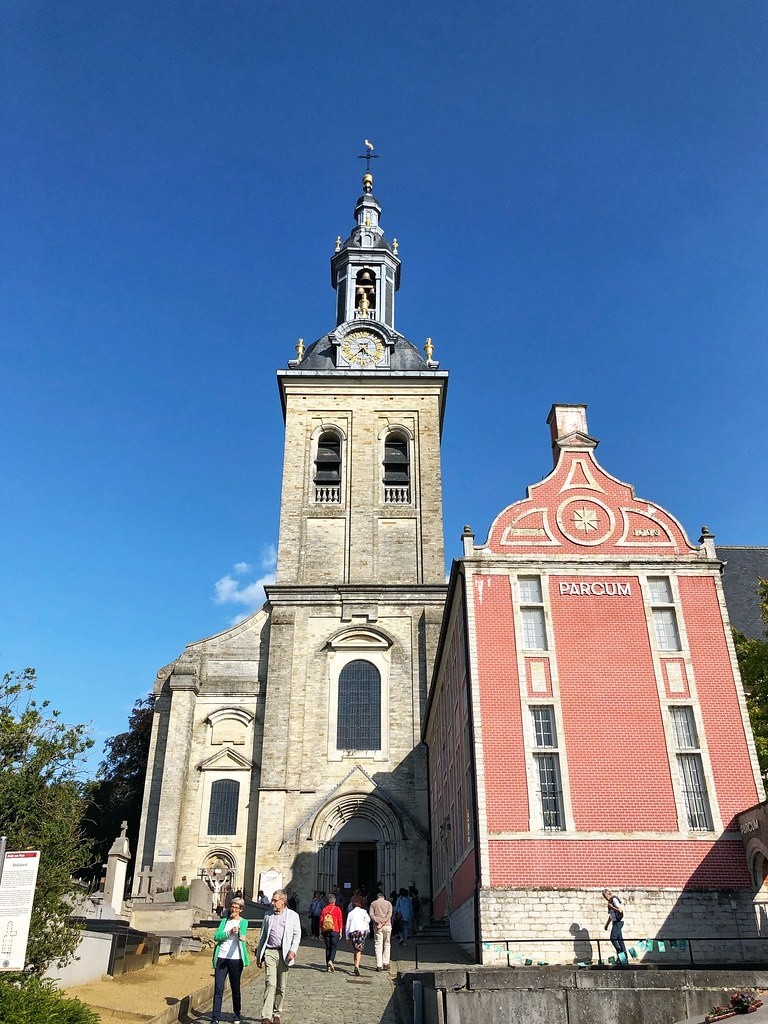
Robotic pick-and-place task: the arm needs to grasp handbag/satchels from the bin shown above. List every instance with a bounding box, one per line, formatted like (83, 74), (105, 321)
(395, 911), (403, 920)
(307, 911), (313, 918)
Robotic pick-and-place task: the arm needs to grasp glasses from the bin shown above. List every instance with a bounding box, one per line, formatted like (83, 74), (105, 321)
(271, 898), (282, 903)
(231, 906), (240, 910)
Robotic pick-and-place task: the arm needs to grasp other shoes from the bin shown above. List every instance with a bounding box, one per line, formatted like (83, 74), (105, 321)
(261, 1018), (272, 1024)
(353, 967), (360, 976)
(210, 1020), (219, 1024)
(399, 938), (403, 945)
(327, 960), (334, 972)
(376, 967), (381, 972)
(404, 943), (408, 946)
(384, 965), (390, 970)
(312, 936), (318, 941)
(233, 1012), (243, 1024)
(273, 1016), (281, 1024)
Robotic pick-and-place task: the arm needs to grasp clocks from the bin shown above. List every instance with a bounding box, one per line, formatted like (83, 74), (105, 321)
(342, 331), (385, 364)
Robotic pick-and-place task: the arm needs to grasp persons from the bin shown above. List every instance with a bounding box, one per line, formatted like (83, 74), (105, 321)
(345, 898), (371, 977)
(258, 890), (269, 905)
(310, 881), (386, 949)
(225, 886), (242, 909)
(368, 893), (393, 971)
(318, 894), (342, 973)
(208, 875), (227, 907)
(257, 889), (301, 1024)
(603, 890), (630, 965)
(394, 890), (414, 946)
(210, 897), (249, 1024)
(285, 887), (299, 914)
(389, 887), (422, 942)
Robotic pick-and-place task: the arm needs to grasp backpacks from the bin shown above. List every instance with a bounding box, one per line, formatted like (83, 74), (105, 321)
(323, 906), (336, 931)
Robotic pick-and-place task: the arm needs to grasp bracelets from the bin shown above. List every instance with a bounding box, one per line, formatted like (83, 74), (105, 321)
(319, 933), (322, 935)
(379, 922), (383, 925)
